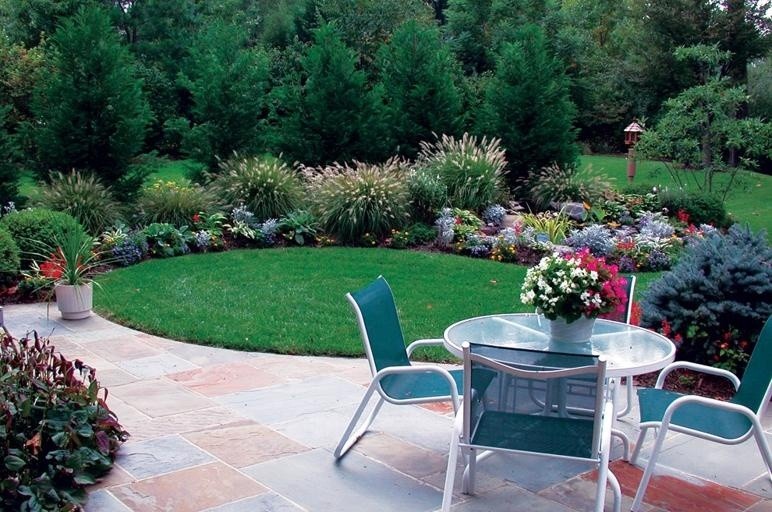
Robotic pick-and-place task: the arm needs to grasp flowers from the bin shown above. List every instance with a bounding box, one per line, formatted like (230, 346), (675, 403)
(519, 247), (630, 317)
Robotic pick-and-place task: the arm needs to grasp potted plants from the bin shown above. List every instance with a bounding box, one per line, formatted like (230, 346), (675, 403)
(10, 209), (137, 320)
